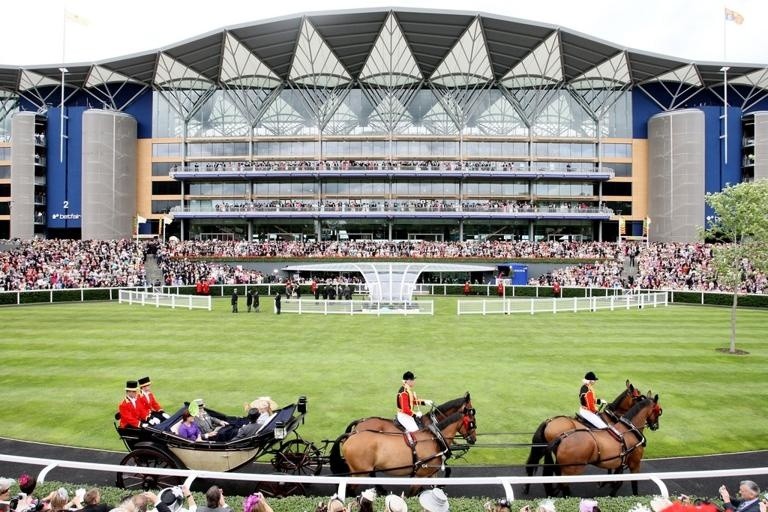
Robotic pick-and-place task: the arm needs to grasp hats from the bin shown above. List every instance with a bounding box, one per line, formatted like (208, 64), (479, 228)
(384, 494), (408, 512)
(419, 488), (449, 512)
(125, 381), (137, 390)
(158, 487), (183, 512)
(137, 376), (150, 387)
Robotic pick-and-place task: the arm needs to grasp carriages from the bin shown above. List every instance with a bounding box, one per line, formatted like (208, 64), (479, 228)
(111, 382), (661, 498)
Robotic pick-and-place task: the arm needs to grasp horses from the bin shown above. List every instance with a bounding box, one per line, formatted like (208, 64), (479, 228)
(344, 389), (473, 491)
(329, 405), (477, 499)
(542, 389), (663, 497)
(522, 379), (644, 494)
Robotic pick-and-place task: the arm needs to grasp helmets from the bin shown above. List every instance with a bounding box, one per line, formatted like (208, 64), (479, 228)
(584, 372), (598, 380)
(402, 372), (416, 380)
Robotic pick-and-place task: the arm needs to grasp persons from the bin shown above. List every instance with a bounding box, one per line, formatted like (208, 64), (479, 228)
(0, 475), (768, 512)
(397, 371), (433, 432)
(0, 239), (768, 315)
(196, 159), (515, 172)
(118, 381), (155, 428)
(138, 377), (171, 424)
(579, 371), (608, 429)
(178, 396), (277, 443)
(217, 199), (607, 213)
